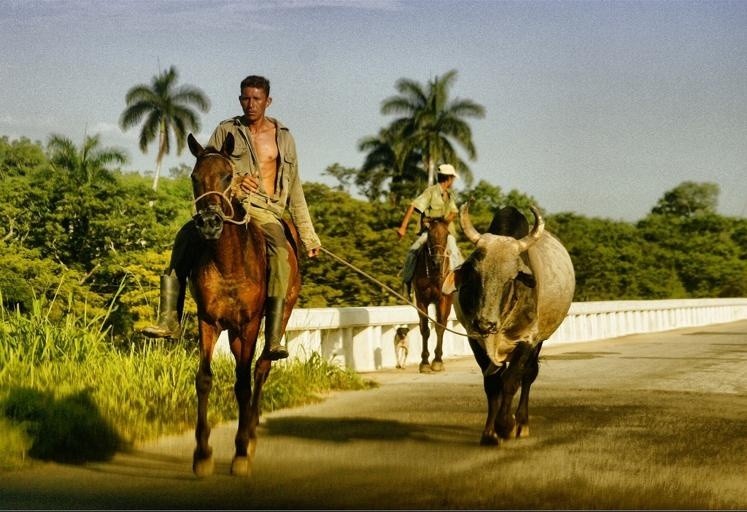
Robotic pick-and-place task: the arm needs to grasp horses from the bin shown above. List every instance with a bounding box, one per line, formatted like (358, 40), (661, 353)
(185, 131), (305, 480)
(411, 210), (456, 377)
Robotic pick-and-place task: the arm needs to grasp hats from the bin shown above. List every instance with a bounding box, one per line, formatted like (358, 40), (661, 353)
(438, 163), (460, 178)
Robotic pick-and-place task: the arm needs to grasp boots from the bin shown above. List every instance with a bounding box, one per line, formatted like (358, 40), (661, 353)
(397, 282), (412, 305)
(142, 275), (187, 340)
(264, 296), (290, 360)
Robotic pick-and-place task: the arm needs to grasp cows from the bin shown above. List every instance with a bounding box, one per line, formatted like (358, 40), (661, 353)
(440, 196), (577, 447)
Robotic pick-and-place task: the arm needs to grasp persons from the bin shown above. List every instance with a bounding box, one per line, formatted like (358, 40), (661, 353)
(143, 76), (320, 359)
(396, 164), (465, 305)
